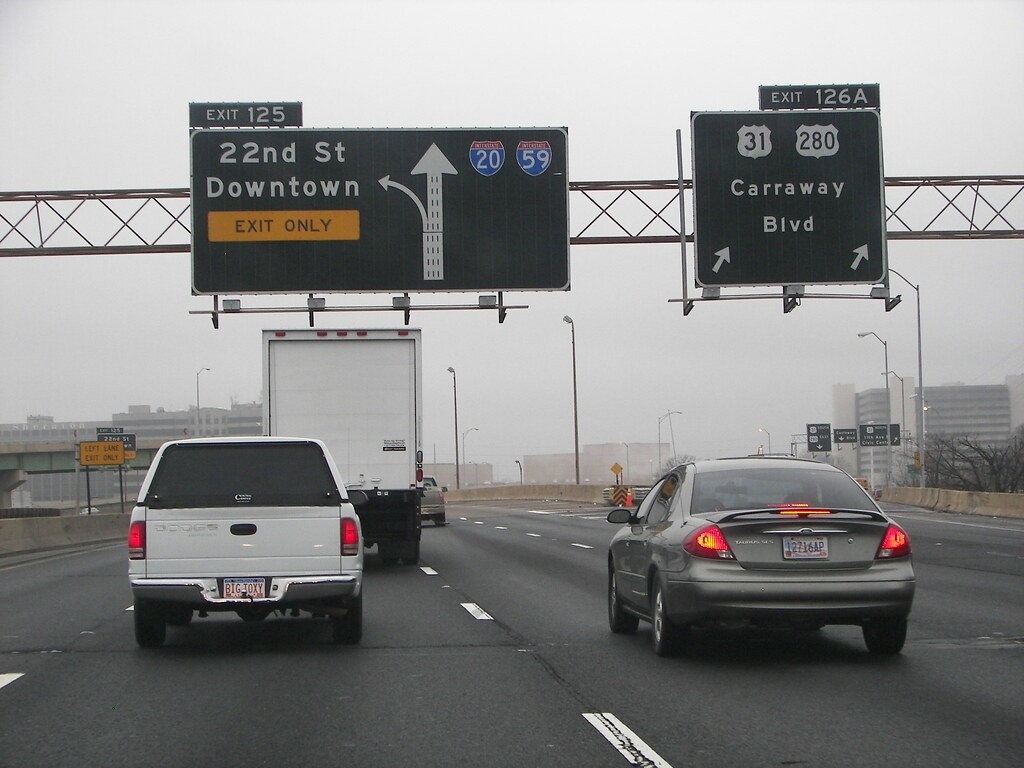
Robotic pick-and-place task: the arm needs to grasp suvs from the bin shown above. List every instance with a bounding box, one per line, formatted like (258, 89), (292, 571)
(420, 476), (448, 525)
(127, 435), (370, 645)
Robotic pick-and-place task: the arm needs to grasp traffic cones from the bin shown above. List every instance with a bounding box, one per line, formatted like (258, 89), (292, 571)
(624, 488), (634, 508)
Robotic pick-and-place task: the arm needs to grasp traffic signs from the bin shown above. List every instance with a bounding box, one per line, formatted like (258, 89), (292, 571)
(187, 126), (573, 297)
(688, 107), (890, 288)
(833, 428), (858, 443)
(859, 423), (900, 446)
(805, 423), (832, 453)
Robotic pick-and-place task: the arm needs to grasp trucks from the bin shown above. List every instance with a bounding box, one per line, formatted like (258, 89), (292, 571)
(259, 327), (425, 565)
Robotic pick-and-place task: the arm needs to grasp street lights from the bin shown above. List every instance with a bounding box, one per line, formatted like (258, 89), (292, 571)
(657, 410), (684, 479)
(881, 370), (907, 486)
(563, 315), (581, 485)
(515, 460), (523, 485)
(857, 330), (892, 487)
(619, 441), (631, 484)
(759, 428), (772, 457)
(196, 367), (211, 436)
(461, 426), (479, 488)
(469, 460), (479, 488)
(446, 367), (461, 490)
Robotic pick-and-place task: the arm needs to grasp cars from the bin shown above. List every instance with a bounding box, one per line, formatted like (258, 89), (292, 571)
(79, 507), (99, 515)
(605, 455), (916, 660)
